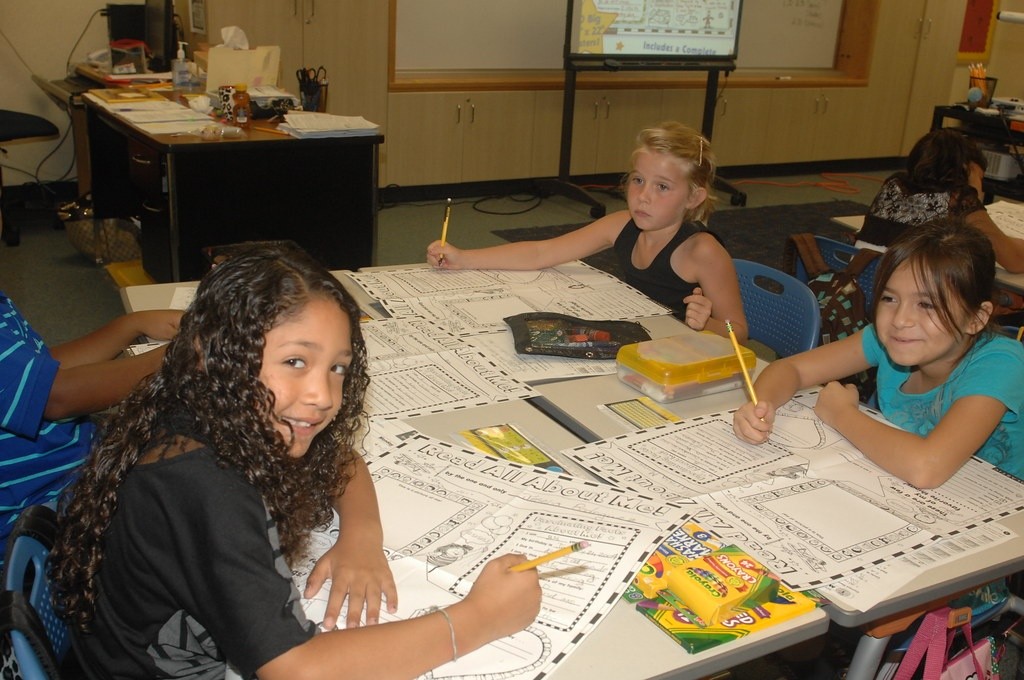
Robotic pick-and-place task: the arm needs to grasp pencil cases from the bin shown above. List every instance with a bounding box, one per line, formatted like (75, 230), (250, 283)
(503, 311), (653, 359)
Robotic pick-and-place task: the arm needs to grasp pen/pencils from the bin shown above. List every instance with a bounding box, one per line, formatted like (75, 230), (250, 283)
(253, 127), (289, 135)
(969, 62), (987, 107)
(724, 319), (765, 422)
(508, 541), (590, 573)
(116, 108), (156, 111)
(530, 320), (641, 358)
(439, 198), (452, 265)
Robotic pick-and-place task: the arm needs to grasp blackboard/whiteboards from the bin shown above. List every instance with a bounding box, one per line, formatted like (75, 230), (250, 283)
(386, 0), (846, 73)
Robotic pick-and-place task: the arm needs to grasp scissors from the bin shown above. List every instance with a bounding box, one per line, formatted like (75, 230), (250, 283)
(296, 64), (326, 111)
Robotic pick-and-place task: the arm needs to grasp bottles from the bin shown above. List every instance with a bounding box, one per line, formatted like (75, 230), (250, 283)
(232, 84), (250, 127)
(188, 74), (202, 100)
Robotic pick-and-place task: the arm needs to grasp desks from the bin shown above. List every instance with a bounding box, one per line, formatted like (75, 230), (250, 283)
(930, 105), (1024, 206)
(31, 63), (385, 283)
(832, 208), (1024, 298)
(121, 255), (1024, 680)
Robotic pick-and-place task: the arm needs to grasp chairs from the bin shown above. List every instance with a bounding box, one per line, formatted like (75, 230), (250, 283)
(795, 233), (879, 313)
(0, 506), (81, 680)
(0, 109), (61, 246)
(866, 325), (1024, 653)
(734, 235), (820, 360)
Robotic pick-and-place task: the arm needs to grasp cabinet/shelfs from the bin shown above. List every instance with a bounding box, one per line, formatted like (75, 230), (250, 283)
(207, 0), (969, 204)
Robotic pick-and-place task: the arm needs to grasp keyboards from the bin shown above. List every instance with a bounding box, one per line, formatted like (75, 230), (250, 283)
(64, 74), (106, 89)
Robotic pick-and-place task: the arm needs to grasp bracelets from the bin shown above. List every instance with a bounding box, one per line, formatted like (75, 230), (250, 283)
(436, 609), (457, 661)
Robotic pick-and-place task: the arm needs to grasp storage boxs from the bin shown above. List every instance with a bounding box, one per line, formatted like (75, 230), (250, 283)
(618, 330), (755, 406)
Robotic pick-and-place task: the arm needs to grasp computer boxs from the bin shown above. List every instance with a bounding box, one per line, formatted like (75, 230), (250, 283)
(107, 4), (177, 60)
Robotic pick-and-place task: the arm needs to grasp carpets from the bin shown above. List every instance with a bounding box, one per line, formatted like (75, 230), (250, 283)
(490, 198), (871, 295)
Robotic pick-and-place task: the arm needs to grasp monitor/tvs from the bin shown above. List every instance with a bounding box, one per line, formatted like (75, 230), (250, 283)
(146, 0), (174, 73)
(563, 1), (743, 61)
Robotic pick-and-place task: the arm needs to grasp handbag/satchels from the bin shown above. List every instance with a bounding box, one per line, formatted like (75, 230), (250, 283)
(895, 607), (1000, 680)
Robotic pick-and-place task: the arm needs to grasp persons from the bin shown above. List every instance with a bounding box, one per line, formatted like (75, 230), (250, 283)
(849, 131), (1024, 273)
(427, 122), (748, 345)
(44, 249), (542, 680)
(734, 218), (1024, 680)
(0, 290), (188, 567)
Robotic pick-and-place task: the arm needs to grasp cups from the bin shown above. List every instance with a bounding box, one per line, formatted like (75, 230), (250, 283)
(218, 85), (236, 119)
(300, 84), (328, 112)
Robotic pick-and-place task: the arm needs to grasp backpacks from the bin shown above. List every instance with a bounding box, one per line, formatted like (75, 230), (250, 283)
(784, 234), (883, 396)
(0, 504), (65, 680)
